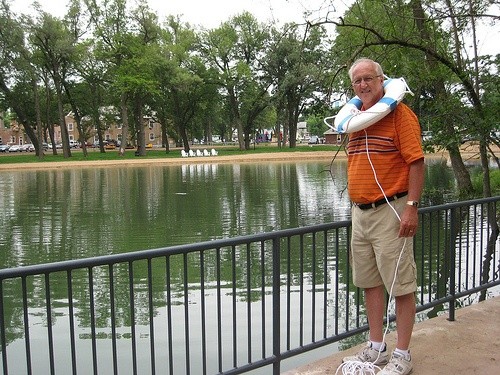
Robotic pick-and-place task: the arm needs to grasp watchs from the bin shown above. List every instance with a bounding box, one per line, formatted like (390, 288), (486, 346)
(407, 201), (419, 207)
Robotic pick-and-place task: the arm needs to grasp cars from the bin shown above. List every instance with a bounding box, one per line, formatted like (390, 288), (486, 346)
(0, 140), (137, 152)
(308, 137), (319, 144)
(421, 130), (500, 148)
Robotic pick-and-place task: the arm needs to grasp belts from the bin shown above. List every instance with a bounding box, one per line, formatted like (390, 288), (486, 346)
(357, 190), (408, 211)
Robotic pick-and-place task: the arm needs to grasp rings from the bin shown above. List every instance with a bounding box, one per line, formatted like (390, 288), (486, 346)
(410, 230), (413, 232)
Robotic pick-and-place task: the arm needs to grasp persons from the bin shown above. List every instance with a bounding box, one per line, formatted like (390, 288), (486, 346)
(345, 58), (426, 375)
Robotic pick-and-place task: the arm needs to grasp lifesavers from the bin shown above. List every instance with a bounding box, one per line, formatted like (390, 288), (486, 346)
(331, 78), (406, 132)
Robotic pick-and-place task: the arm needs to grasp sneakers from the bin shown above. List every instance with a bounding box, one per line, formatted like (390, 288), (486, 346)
(376, 350), (414, 375)
(342, 342), (389, 366)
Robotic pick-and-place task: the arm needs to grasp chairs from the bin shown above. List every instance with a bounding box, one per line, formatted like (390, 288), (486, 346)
(180, 148), (219, 157)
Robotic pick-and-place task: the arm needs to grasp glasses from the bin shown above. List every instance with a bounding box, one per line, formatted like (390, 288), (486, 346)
(351, 74), (382, 85)
